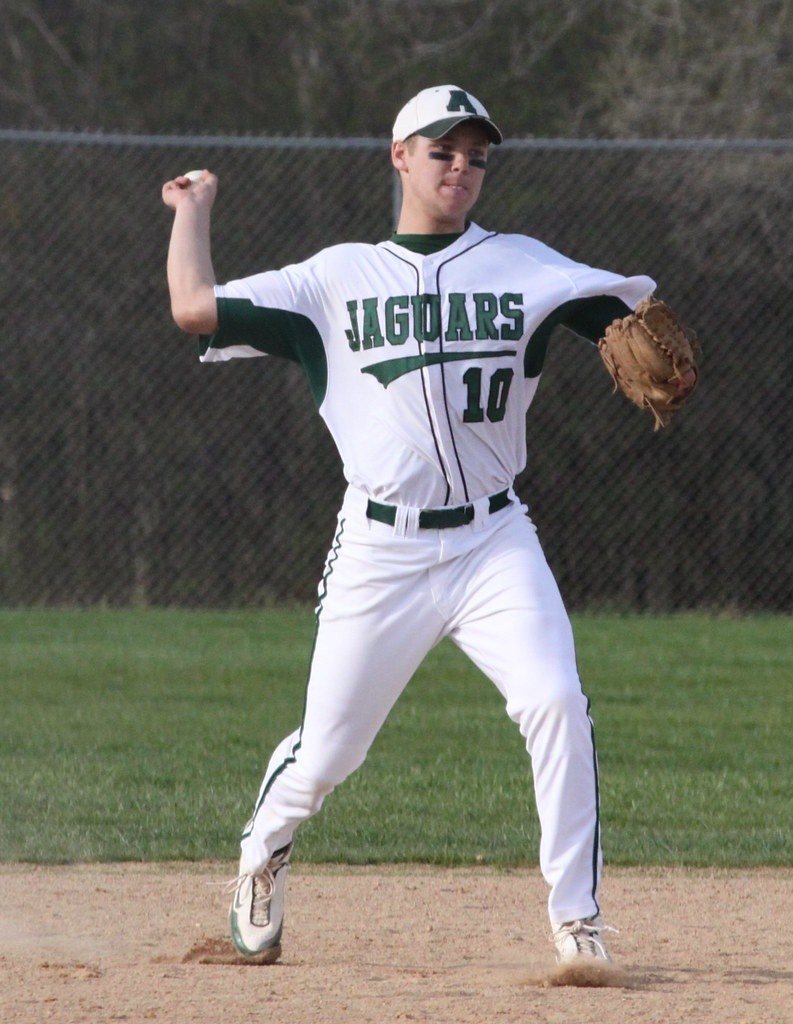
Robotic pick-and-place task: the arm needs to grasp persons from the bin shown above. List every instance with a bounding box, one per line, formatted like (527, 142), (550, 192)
(163, 83), (697, 967)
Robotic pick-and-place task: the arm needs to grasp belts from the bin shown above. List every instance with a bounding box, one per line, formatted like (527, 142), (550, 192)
(366, 488), (512, 528)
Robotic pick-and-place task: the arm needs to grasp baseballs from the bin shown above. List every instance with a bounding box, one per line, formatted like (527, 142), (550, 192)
(184, 169), (205, 189)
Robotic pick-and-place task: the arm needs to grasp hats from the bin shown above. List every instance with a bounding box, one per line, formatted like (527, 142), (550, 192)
(393, 85), (503, 146)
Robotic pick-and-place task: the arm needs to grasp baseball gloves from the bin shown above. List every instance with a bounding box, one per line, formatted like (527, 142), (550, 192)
(597, 294), (701, 432)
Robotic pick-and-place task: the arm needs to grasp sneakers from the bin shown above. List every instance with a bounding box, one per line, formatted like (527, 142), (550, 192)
(206, 841), (294, 958)
(549, 914), (621, 972)
